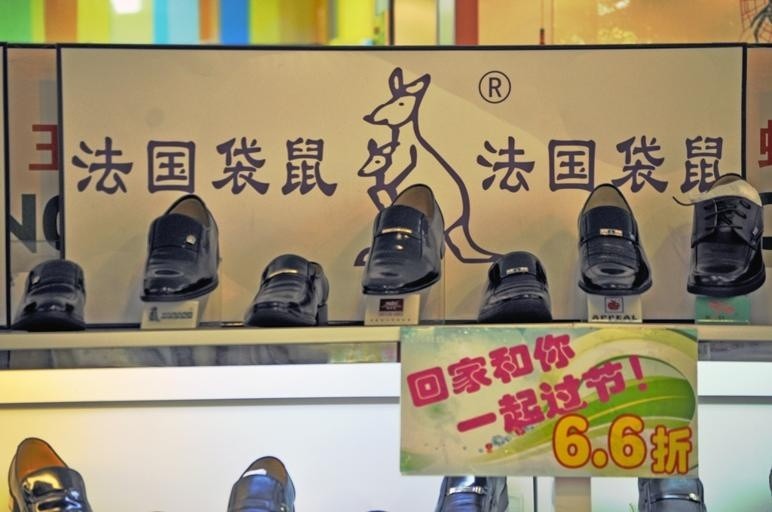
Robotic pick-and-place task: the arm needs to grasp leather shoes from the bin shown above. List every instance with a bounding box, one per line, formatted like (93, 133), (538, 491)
(435, 476), (510, 512)
(243, 254), (330, 328)
(8, 436), (92, 512)
(227, 456), (296, 512)
(638, 477), (707, 512)
(576, 182), (653, 297)
(361, 183), (446, 296)
(138, 194), (220, 303)
(477, 250), (552, 322)
(684, 173), (766, 299)
(12, 258), (87, 331)
